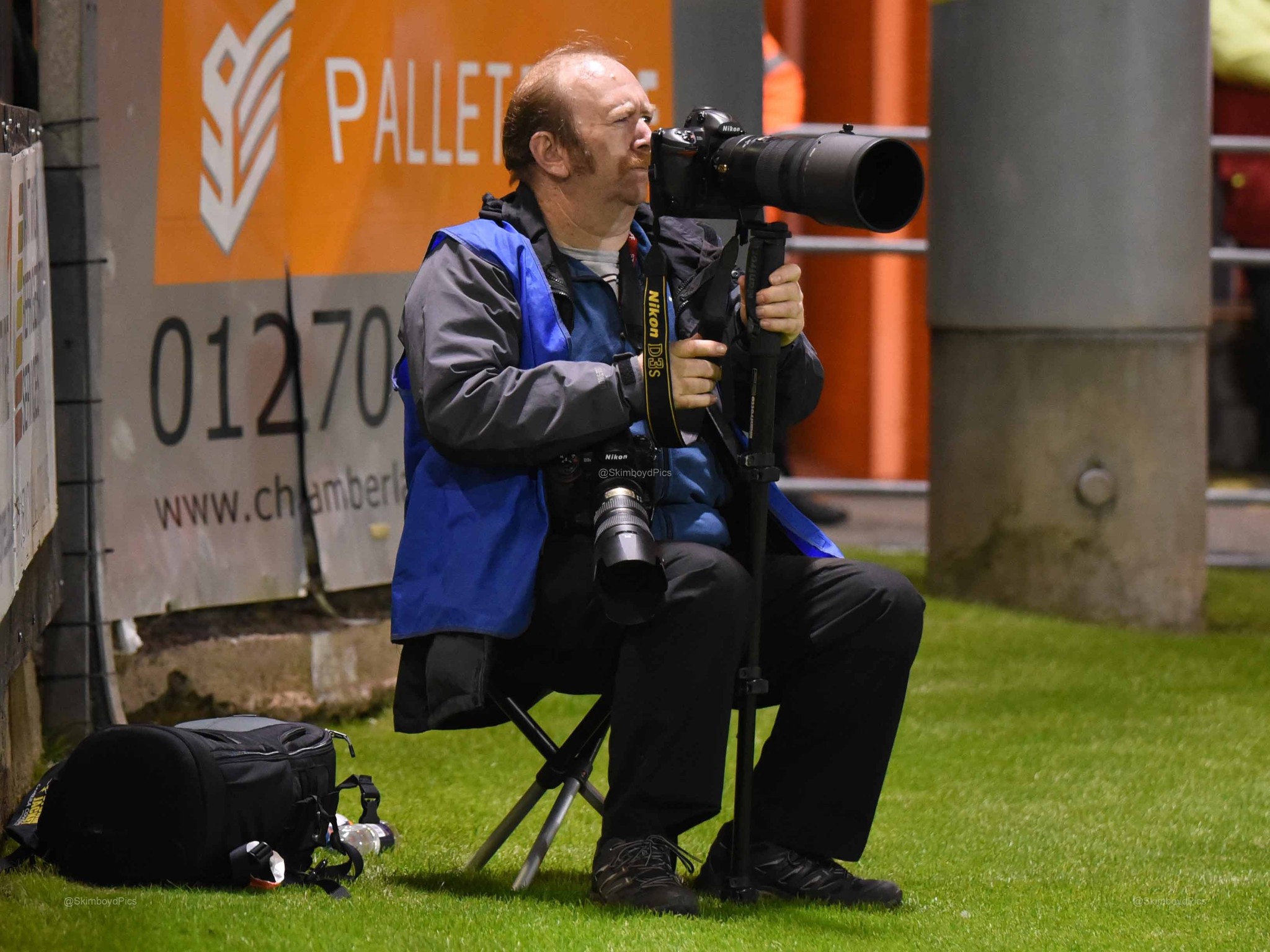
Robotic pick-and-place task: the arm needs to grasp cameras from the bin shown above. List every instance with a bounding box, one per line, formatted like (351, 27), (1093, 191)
(651, 105), (925, 234)
(543, 427), (671, 625)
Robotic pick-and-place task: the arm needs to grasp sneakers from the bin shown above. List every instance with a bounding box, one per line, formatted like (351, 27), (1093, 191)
(696, 820), (902, 907)
(587, 835), (700, 915)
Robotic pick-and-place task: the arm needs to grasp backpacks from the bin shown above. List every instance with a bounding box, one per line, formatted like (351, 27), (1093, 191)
(1, 713), (396, 895)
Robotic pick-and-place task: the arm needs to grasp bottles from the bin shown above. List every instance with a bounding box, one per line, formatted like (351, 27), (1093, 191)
(328, 820), (403, 856)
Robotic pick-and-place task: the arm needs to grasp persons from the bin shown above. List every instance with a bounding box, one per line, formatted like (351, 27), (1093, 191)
(388, 43), (927, 908)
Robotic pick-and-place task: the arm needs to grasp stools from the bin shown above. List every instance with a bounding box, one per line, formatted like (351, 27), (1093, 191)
(462, 657), (784, 894)
